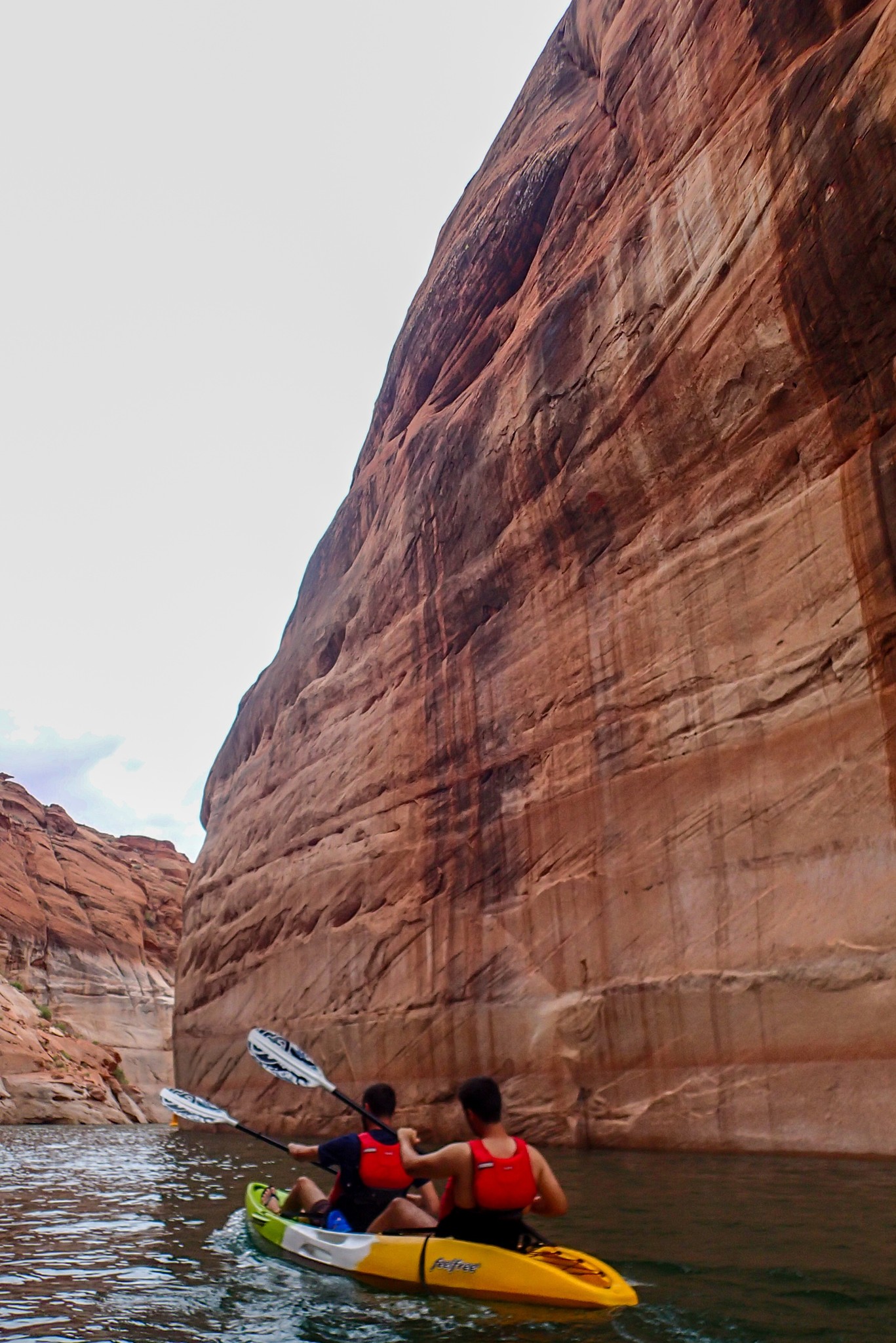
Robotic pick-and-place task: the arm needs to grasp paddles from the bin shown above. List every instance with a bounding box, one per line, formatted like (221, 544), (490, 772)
(246, 1025), (559, 1249)
(158, 1087), (339, 1175)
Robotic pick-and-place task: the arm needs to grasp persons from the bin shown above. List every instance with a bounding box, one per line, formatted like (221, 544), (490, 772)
(260, 1082), (440, 1235)
(326, 1074), (568, 1252)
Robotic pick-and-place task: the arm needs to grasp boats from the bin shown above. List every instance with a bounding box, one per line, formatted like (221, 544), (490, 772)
(244, 1182), (639, 1309)
